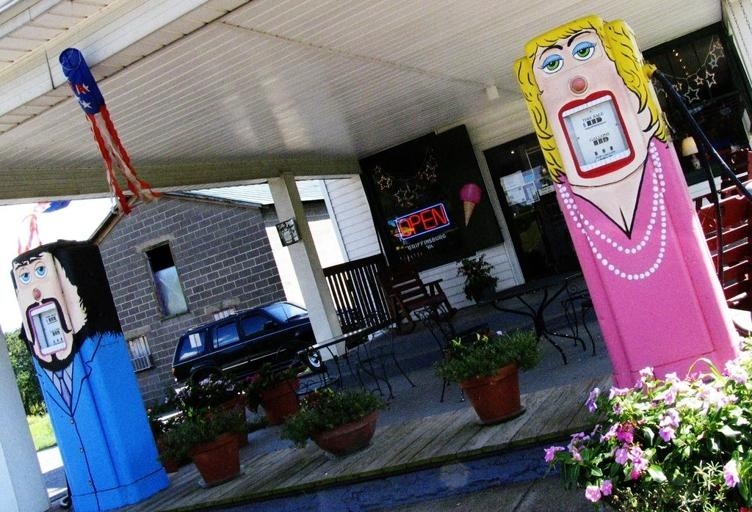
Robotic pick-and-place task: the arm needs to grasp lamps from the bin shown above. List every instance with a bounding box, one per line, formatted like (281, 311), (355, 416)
(680, 132), (702, 170)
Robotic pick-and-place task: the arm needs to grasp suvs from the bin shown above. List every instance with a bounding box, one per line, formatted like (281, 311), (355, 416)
(171, 300), (366, 399)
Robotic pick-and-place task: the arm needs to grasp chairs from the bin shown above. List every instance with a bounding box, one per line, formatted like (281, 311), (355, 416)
(692, 181), (751, 311)
(561, 272), (597, 358)
(411, 289), (484, 405)
(375, 258), (457, 335)
(719, 146), (752, 198)
(354, 312), (416, 399)
(269, 337), (345, 407)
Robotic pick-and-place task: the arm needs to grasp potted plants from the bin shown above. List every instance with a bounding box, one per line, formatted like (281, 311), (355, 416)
(273, 377), (393, 463)
(143, 355), (299, 466)
(161, 407), (257, 481)
(426, 324), (549, 424)
(453, 252), (499, 303)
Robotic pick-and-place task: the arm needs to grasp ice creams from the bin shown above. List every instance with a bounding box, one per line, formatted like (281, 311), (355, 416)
(459, 183), (483, 228)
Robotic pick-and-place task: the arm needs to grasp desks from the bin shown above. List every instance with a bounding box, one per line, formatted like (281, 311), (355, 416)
(302, 317), (421, 408)
(488, 272), (587, 364)
